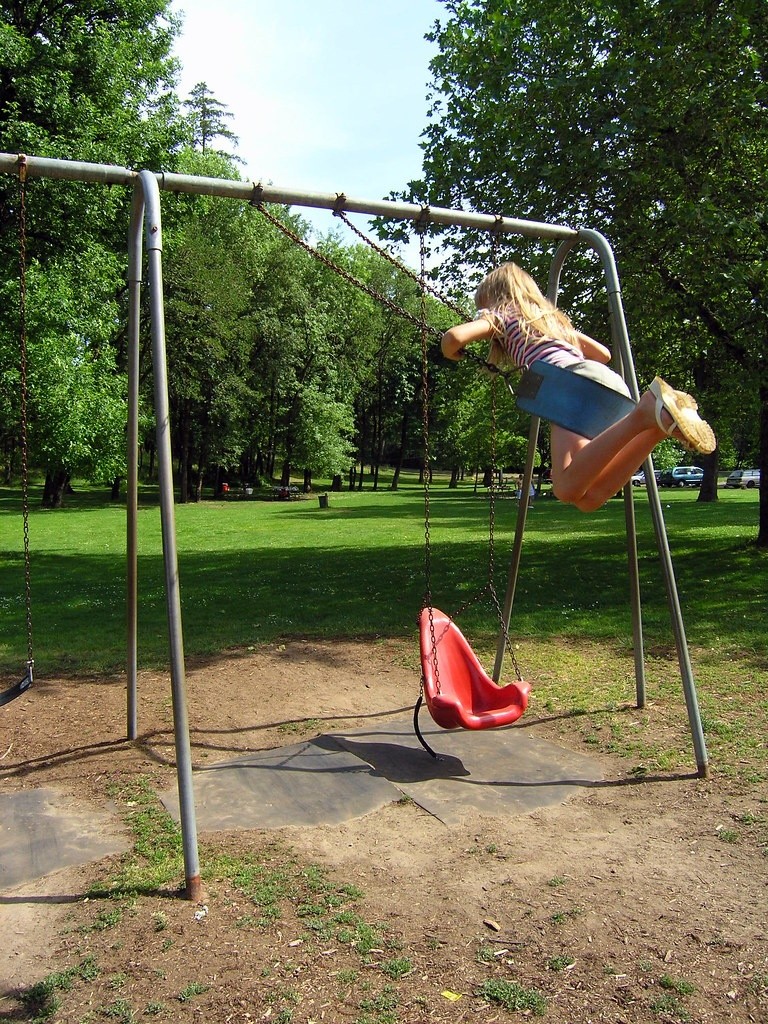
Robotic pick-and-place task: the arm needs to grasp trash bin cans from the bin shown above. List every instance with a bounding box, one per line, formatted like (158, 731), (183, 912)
(318, 496), (328, 508)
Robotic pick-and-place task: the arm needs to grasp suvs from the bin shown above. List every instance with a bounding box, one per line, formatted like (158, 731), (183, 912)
(542, 469), (553, 484)
(726, 469), (761, 488)
(631, 470), (661, 485)
(660, 466), (705, 488)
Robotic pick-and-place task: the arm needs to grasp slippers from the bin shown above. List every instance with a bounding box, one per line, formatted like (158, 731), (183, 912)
(674, 390), (716, 454)
(648, 376), (701, 450)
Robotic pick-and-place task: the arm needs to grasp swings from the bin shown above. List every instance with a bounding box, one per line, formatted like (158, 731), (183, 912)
(256, 206), (637, 441)
(418, 235), (531, 734)
(0, 183), (35, 707)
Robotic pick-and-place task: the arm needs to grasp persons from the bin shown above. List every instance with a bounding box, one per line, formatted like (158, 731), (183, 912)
(440, 261), (718, 515)
(528, 482), (537, 509)
(514, 475), (523, 508)
